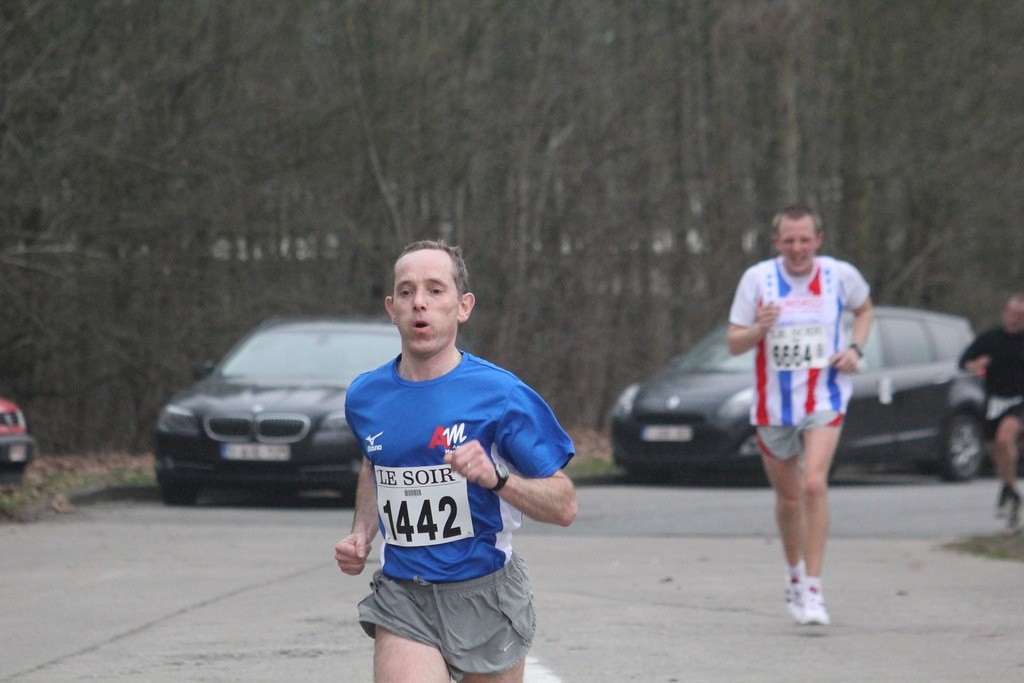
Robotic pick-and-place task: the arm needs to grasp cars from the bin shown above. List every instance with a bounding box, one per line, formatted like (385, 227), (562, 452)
(153, 317), (403, 508)
(0, 401), (37, 483)
(606, 305), (988, 489)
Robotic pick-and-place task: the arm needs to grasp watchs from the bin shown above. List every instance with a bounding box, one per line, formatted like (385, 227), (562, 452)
(488, 462), (509, 494)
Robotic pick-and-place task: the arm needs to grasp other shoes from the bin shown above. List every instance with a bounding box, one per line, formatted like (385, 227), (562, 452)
(1008, 498), (1024, 533)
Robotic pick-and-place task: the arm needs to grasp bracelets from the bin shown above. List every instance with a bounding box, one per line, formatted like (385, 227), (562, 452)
(849, 343), (863, 359)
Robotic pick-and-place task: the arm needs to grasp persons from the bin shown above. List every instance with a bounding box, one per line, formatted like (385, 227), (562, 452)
(959, 291), (1024, 527)
(336, 239), (577, 683)
(728, 203), (871, 626)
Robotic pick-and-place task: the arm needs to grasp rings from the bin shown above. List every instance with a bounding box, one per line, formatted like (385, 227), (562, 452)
(467, 461), (470, 471)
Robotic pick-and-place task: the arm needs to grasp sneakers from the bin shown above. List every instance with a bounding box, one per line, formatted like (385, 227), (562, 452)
(785, 577), (829, 625)
